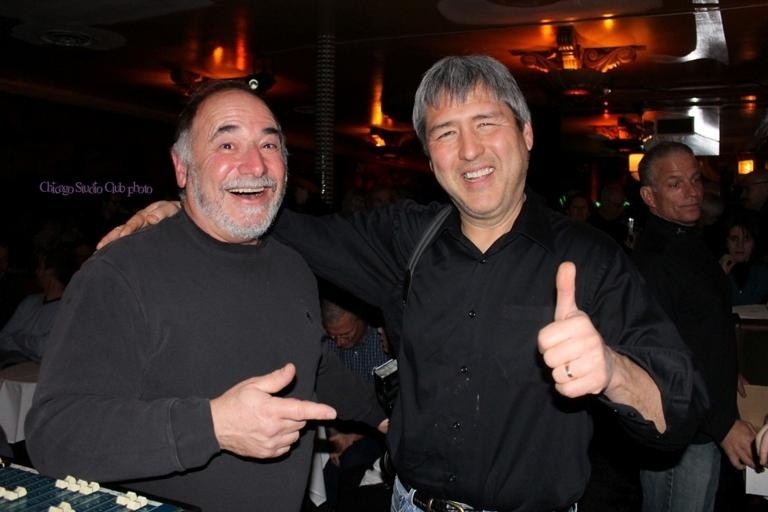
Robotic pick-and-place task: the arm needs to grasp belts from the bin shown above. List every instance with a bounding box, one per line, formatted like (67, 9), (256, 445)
(395, 471), (487, 512)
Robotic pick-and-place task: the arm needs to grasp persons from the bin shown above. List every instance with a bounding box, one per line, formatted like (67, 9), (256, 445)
(0, 55), (768, 512)
